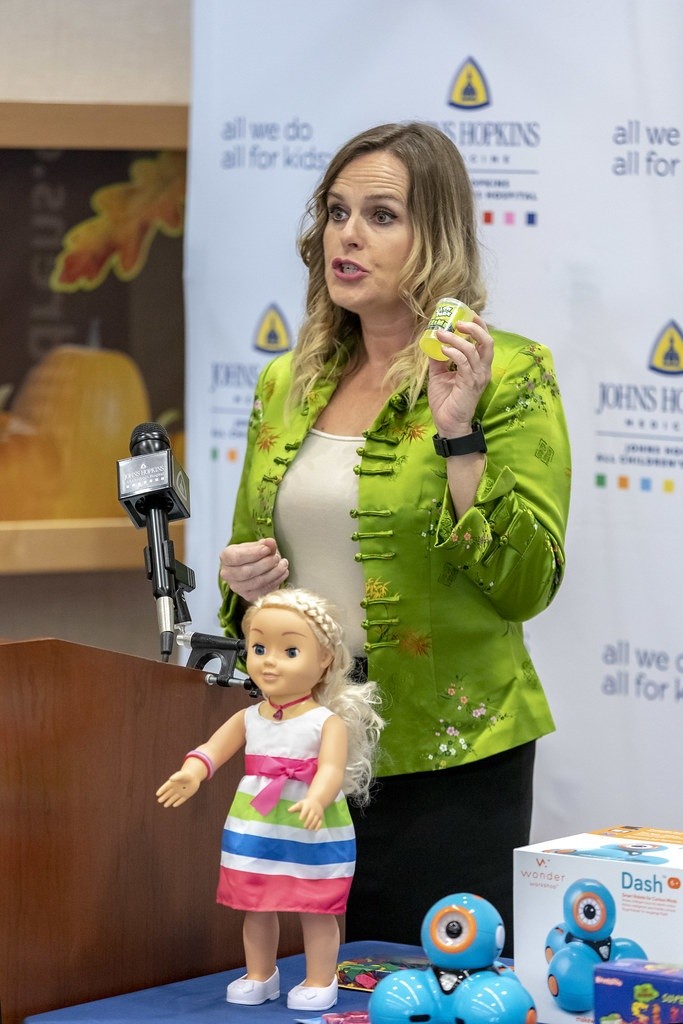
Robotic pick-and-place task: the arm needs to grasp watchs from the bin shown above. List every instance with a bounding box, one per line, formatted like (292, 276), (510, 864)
(431, 421), (488, 457)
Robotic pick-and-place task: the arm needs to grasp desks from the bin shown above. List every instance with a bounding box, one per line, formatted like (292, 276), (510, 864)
(22, 939), (517, 1024)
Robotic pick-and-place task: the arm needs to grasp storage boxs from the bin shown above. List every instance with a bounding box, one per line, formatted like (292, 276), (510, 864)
(592, 957), (682, 1024)
(513, 824), (682, 1024)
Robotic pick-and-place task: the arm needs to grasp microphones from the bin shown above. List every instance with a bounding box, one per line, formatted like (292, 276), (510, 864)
(129, 421), (184, 656)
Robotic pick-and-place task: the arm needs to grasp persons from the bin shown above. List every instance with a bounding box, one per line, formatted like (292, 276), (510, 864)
(156, 589), (389, 1011)
(218, 120), (573, 962)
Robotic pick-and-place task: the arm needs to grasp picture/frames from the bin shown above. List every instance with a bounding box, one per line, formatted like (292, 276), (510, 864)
(0, 102), (191, 577)
